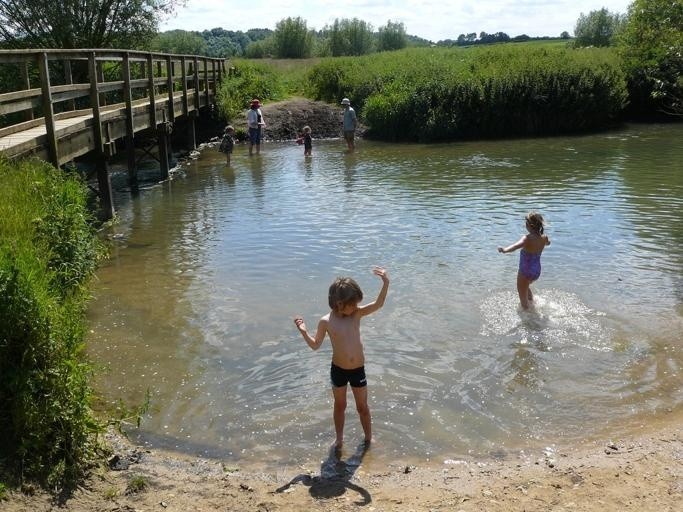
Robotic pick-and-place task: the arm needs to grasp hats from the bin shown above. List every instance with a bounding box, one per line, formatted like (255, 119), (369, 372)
(304, 125), (311, 132)
(340, 98), (349, 105)
(249, 100), (261, 105)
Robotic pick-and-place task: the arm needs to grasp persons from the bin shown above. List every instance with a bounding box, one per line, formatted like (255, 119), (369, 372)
(247, 98), (266, 156)
(498, 212), (551, 309)
(218, 126), (240, 163)
(341, 98), (358, 148)
(294, 267), (389, 449)
(302, 126), (312, 156)
